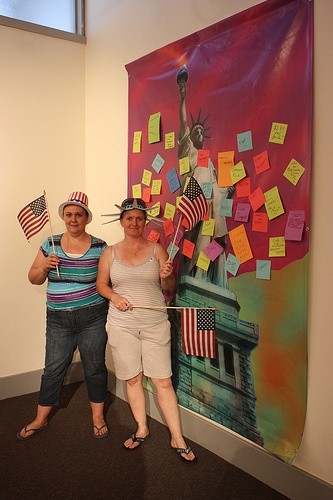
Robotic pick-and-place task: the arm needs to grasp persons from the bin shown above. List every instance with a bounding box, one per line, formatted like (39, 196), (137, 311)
(95, 197), (198, 463)
(16, 191), (110, 439)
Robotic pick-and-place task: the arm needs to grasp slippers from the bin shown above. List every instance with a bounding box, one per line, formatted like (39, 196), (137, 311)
(93, 425), (109, 439)
(123, 433), (150, 450)
(17, 422), (48, 441)
(171, 446), (197, 462)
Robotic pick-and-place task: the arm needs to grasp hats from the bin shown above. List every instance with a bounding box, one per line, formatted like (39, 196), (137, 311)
(58, 192), (93, 225)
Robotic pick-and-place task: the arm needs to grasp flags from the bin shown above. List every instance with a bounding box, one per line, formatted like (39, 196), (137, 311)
(17, 195), (51, 243)
(179, 307), (216, 360)
(176, 176), (208, 232)
